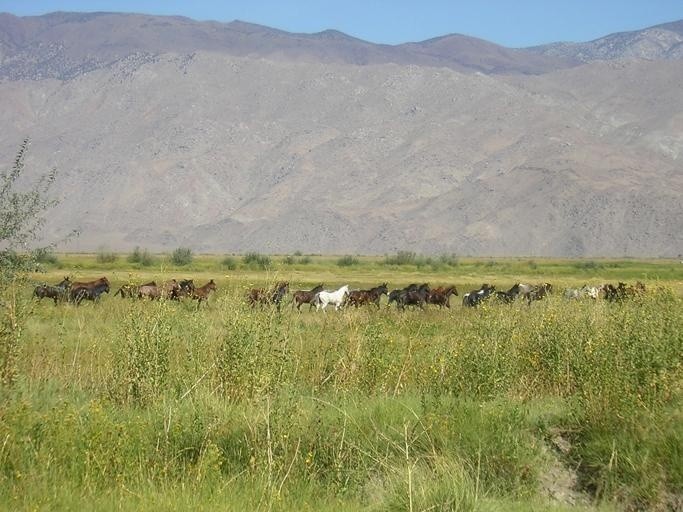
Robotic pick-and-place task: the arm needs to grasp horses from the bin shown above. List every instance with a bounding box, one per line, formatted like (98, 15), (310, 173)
(33, 276), (219, 312)
(461, 279), (647, 311)
(243, 279), (459, 316)
